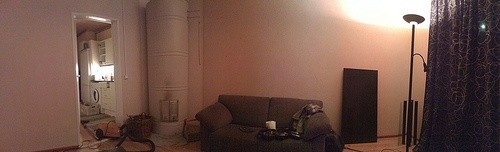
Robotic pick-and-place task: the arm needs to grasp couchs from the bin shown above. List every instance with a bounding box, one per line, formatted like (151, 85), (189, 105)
(195, 94), (344, 152)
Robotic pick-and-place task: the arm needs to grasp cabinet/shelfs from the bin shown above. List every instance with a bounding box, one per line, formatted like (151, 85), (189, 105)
(88, 82), (116, 118)
(97, 37), (114, 66)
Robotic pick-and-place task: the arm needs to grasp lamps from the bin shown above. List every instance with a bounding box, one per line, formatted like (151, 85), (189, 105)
(404, 14), (427, 152)
